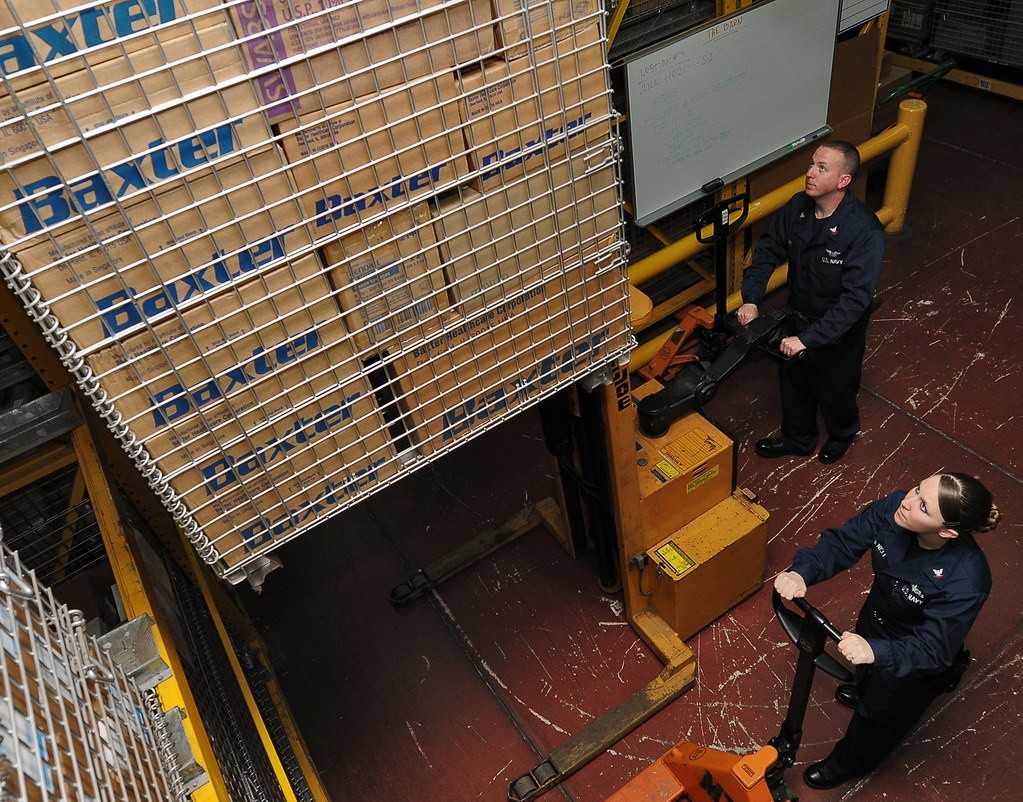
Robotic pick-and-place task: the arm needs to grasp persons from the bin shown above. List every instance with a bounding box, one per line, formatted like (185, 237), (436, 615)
(775, 472), (1001, 790)
(737, 140), (885, 464)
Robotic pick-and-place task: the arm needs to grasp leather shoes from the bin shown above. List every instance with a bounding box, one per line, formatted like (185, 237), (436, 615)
(755, 435), (815, 457)
(803, 759), (865, 790)
(835, 683), (860, 710)
(818, 425), (860, 464)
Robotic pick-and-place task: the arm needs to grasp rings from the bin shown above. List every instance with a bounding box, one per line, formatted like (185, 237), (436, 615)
(787, 353), (790, 354)
(850, 653), (854, 659)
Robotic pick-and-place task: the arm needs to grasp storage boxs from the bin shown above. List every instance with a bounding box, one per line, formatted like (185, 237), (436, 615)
(873, 65), (913, 123)
(0, 0), (632, 802)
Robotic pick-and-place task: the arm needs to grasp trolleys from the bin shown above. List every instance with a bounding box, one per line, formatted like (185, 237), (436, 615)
(605, 589), (865, 802)
